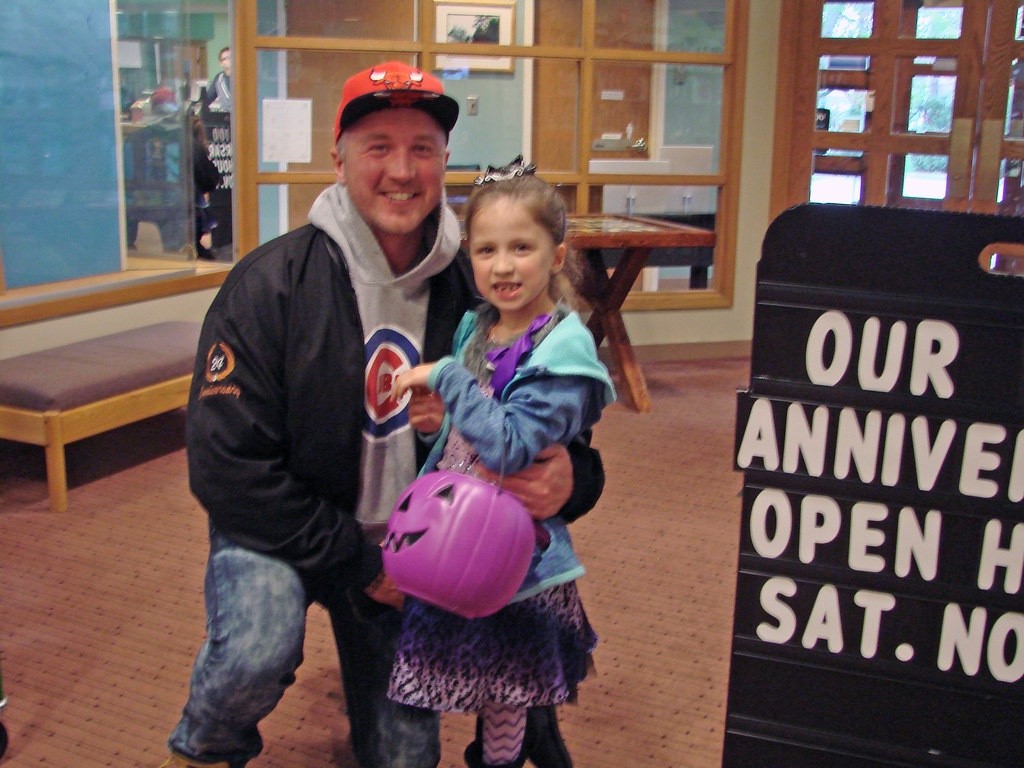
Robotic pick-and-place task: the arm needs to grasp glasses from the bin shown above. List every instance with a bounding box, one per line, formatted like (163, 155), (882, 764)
(220, 56), (231, 62)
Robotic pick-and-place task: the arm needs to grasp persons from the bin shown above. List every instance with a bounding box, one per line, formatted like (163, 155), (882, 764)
(383, 174), (605, 768)
(117, 45), (232, 260)
(164, 63), (606, 768)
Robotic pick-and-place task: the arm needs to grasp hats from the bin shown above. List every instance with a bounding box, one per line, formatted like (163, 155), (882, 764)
(334, 61), (459, 148)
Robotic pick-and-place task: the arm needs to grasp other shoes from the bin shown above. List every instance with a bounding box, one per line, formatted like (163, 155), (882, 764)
(157, 739), (263, 768)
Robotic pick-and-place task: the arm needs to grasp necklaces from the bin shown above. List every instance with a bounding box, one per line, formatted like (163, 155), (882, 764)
(490, 326), (495, 344)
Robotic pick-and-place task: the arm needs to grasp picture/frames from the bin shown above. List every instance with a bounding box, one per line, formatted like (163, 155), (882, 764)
(432, 1), (519, 74)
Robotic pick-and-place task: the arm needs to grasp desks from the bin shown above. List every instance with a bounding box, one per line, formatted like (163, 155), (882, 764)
(449, 212), (717, 414)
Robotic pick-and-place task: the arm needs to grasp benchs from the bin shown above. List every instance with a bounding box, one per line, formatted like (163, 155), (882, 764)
(0, 321), (204, 513)
